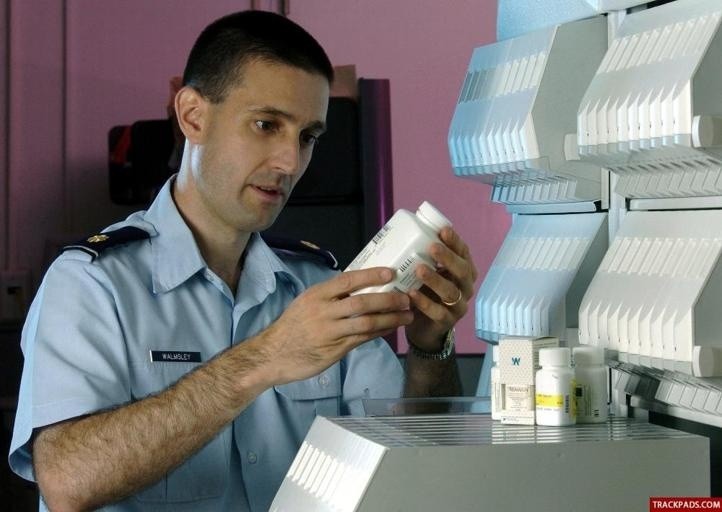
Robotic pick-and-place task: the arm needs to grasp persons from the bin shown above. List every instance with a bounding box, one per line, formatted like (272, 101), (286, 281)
(8, 9), (479, 512)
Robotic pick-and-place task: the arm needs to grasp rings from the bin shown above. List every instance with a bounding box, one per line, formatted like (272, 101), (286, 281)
(442, 290), (462, 307)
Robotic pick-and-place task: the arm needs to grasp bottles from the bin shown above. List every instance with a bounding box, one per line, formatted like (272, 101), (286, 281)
(489, 345), (502, 420)
(572, 345), (608, 425)
(342, 200), (454, 297)
(534, 348), (574, 428)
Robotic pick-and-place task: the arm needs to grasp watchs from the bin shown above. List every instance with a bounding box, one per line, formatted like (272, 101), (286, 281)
(407, 328), (456, 360)
